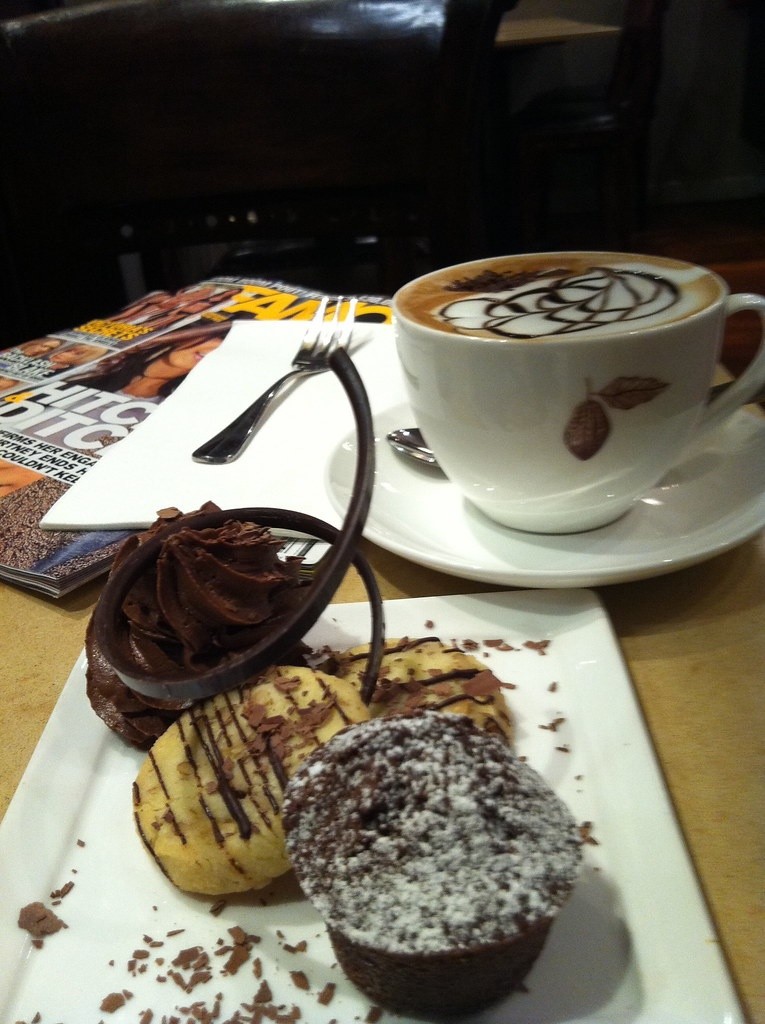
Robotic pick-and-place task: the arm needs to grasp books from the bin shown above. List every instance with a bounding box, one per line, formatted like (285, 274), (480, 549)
(0, 273), (394, 595)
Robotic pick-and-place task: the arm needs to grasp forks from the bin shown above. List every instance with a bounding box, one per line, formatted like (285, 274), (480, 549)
(192, 296), (357, 465)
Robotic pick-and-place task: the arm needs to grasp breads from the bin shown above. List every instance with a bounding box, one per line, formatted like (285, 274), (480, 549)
(81, 347), (385, 748)
(130, 665), (373, 896)
(326, 638), (510, 749)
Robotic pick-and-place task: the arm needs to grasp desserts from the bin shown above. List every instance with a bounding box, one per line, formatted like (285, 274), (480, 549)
(280, 711), (583, 1018)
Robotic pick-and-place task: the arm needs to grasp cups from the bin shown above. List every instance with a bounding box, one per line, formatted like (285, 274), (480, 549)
(391, 251), (765, 534)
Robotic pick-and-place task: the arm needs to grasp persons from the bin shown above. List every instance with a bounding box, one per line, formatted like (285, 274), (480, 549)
(19, 338), (61, 359)
(64, 318), (233, 406)
(107, 287), (243, 332)
(42, 344), (108, 378)
(0, 376), (18, 390)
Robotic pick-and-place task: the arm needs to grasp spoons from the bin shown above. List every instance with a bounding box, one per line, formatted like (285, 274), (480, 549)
(385, 427), (440, 470)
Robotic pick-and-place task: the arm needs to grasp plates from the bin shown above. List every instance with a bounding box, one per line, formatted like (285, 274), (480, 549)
(326, 394), (765, 590)
(1, 589), (746, 1024)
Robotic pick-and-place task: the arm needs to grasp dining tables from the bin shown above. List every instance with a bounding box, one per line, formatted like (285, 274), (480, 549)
(0, 363), (765, 1024)
